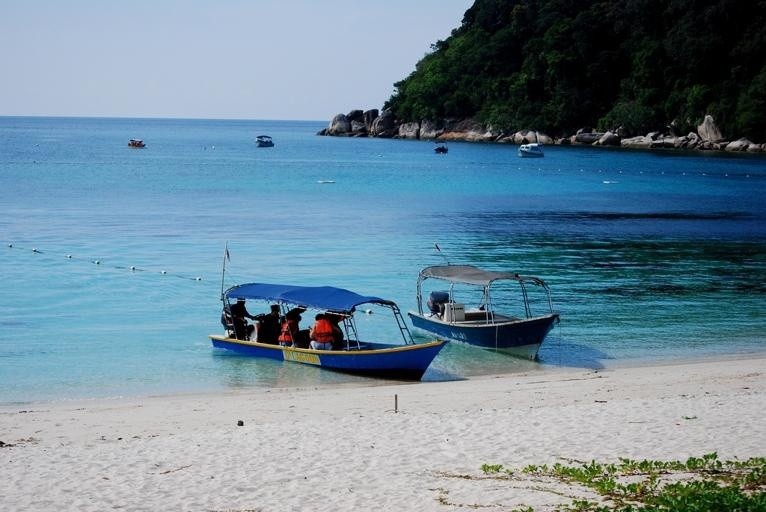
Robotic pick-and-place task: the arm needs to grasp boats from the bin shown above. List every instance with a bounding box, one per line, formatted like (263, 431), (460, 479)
(207, 242), (449, 383)
(433, 140), (448, 153)
(407, 245), (560, 364)
(127, 137), (144, 148)
(520, 142), (544, 157)
(254, 135), (273, 147)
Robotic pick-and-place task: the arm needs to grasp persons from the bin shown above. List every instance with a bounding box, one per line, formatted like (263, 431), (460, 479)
(263, 304), (283, 346)
(285, 305), (311, 346)
(308, 314), (340, 350)
(325, 305), (355, 351)
(220, 296), (265, 341)
(278, 314), (306, 348)
